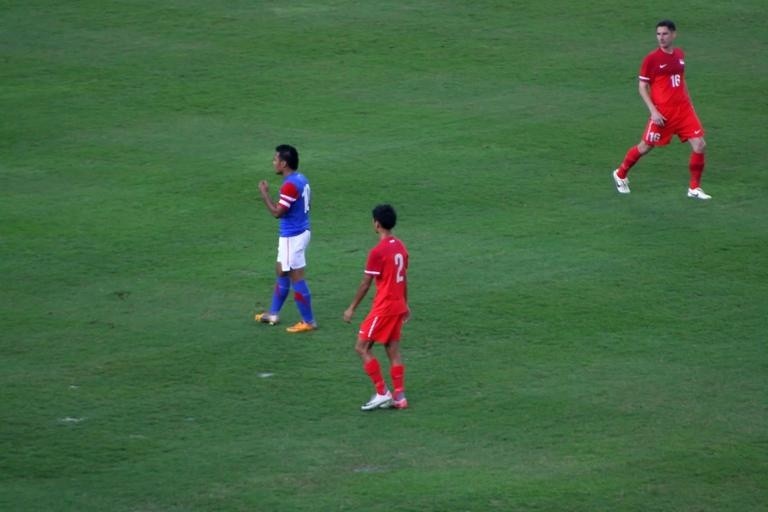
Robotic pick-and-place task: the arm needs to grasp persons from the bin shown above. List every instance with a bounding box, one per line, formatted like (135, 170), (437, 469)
(253, 144), (320, 333)
(340, 202), (413, 412)
(611, 19), (715, 201)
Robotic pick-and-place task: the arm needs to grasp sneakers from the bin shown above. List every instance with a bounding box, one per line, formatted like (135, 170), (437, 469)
(255, 312), (280, 325)
(687, 187), (713, 200)
(360, 390), (409, 411)
(612, 168), (632, 195)
(286, 320), (318, 333)
